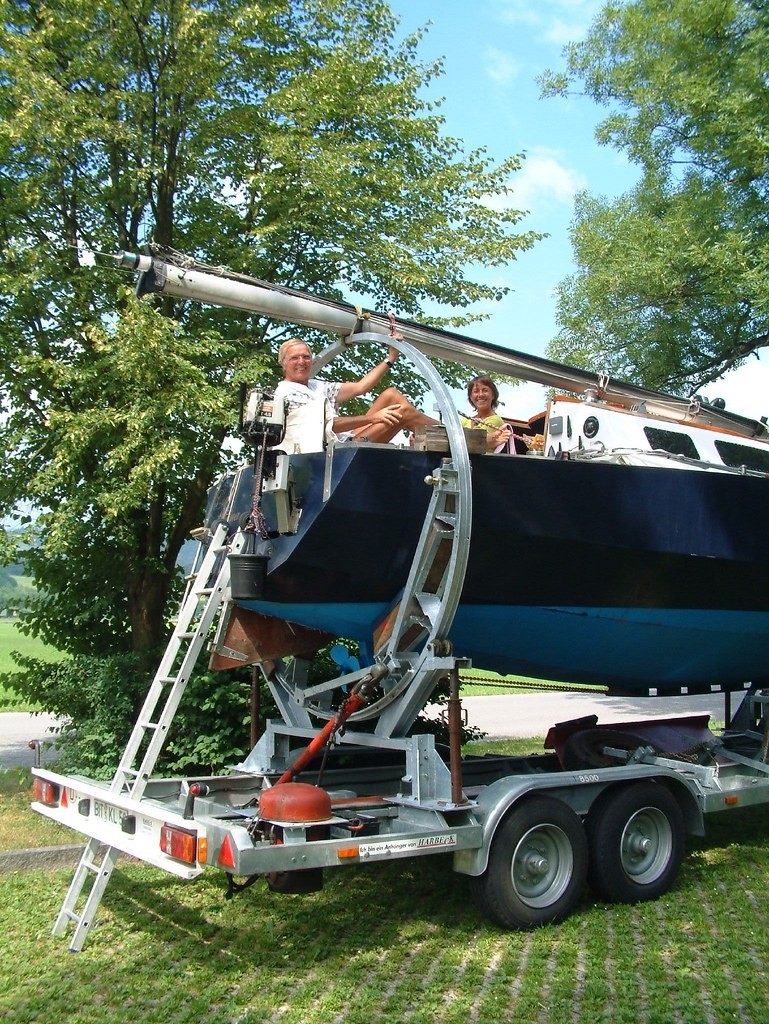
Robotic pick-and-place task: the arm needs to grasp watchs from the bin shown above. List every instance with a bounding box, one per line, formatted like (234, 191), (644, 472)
(384, 358), (393, 368)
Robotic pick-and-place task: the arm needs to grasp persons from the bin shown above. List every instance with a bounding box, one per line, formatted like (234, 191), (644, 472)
(273, 334), (511, 456)
(410, 375), (505, 454)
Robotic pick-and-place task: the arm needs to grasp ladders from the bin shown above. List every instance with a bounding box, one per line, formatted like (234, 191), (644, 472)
(47, 465), (256, 952)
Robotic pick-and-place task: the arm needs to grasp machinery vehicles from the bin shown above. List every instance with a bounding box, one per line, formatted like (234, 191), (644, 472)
(30, 247), (769, 930)
